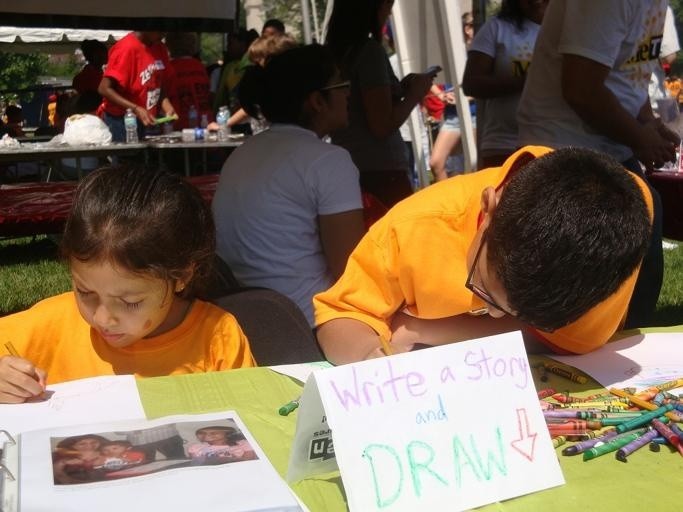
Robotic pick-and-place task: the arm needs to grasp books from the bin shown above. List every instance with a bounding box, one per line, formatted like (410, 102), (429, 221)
(398, 65), (441, 97)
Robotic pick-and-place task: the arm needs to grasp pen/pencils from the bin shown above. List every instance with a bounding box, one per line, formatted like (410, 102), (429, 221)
(537, 377), (683, 460)
(278, 398), (300, 416)
(378, 334), (392, 355)
(535, 360), (587, 383)
(3, 340), (46, 401)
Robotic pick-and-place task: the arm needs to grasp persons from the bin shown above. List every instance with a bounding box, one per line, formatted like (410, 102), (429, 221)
(514, 0), (681, 332)
(101, 441), (148, 465)
(189, 426), (257, 465)
(462, 0), (552, 170)
(326, 0), (437, 212)
(0, 22), (295, 168)
(419, 12), (481, 183)
(205, 44), (366, 334)
(647, 61), (666, 119)
(310, 145), (656, 367)
(0, 162), (260, 403)
(663, 72), (683, 112)
(52, 435), (109, 485)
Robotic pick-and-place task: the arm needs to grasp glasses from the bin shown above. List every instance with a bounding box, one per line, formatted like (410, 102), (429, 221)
(321, 79), (351, 91)
(464, 229), (552, 335)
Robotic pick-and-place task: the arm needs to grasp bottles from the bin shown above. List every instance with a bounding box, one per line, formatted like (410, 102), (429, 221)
(124, 108), (139, 143)
(217, 105), (232, 145)
(163, 120), (175, 134)
(181, 105), (211, 142)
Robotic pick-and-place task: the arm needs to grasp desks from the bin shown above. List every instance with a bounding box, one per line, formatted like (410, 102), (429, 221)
(1, 139), (150, 180)
(150, 137), (244, 176)
(0, 323), (683, 512)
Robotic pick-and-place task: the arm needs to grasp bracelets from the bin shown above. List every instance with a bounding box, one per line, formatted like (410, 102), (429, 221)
(399, 96), (414, 111)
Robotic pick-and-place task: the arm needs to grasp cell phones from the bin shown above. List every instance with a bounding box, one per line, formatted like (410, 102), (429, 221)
(424, 65), (442, 78)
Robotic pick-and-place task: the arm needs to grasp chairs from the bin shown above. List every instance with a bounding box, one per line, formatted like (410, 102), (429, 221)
(204, 286), (320, 365)
